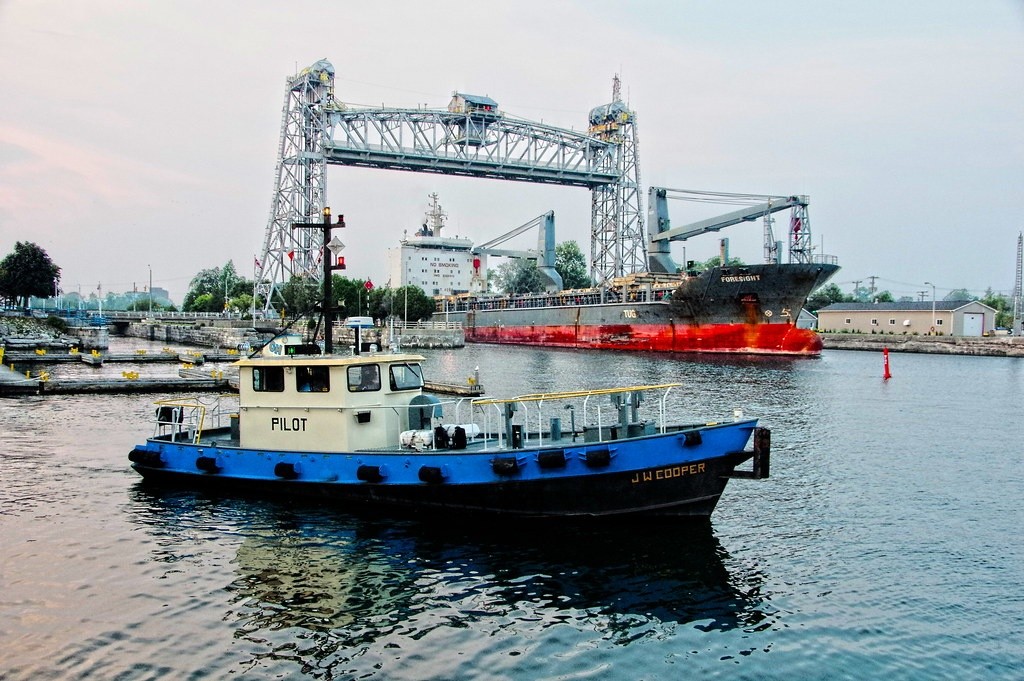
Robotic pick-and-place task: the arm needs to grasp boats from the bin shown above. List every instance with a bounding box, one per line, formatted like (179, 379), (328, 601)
(126, 205), (771, 522)
(423, 187), (841, 354)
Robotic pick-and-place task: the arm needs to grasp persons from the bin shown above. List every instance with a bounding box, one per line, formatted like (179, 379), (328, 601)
(448, 426), (467, 450)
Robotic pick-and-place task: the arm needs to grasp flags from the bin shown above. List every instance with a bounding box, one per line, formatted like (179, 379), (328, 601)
(256, 259), (263, 270)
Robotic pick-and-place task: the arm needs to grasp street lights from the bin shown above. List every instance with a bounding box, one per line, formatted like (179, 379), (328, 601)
(925, 282), (935, 328)
